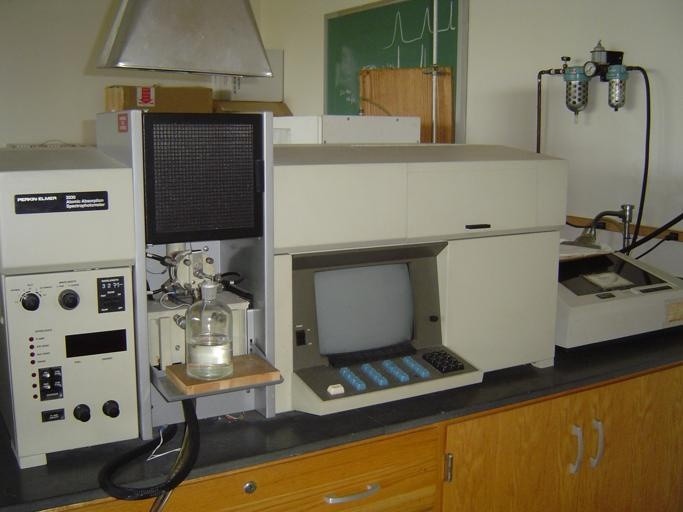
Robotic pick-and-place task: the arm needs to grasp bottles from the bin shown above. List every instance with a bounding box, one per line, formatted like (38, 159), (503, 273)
(184, 281), (234, 382)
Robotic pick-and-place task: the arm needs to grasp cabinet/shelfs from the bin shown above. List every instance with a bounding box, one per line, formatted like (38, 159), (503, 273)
(56, 411), (441, 510)
(441, 361), (682, 509)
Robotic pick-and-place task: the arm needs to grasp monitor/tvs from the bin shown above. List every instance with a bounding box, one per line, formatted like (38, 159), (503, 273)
(292, 242), (448, 370)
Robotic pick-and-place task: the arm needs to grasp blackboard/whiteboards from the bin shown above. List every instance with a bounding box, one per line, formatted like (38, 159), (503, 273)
(323, 0), (469, 145)
